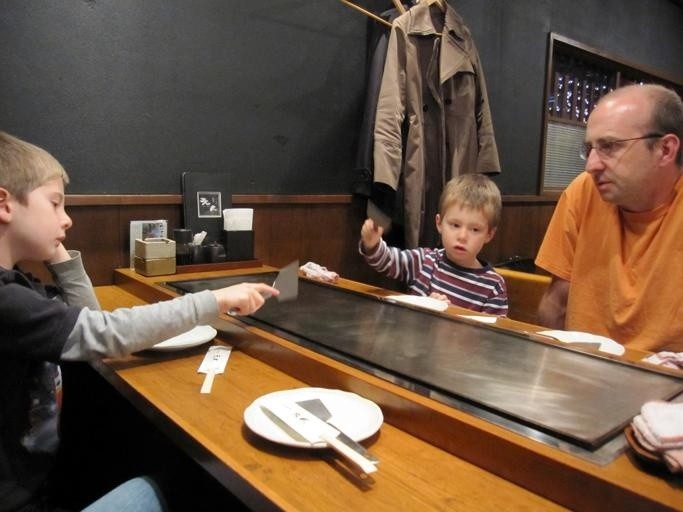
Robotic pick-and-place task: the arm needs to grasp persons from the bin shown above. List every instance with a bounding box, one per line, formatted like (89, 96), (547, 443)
(1, 128), (281, 512)
(535, 82), (683, 357)
(358, 172), (510, 318)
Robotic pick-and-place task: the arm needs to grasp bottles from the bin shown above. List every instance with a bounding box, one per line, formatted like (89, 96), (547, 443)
(173, 228), (226, 266)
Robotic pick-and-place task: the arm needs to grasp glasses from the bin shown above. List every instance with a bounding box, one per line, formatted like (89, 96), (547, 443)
(579, 134), (664, 160)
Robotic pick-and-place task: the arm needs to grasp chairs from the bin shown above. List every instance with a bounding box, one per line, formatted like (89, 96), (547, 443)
(491, 265), (553, 323)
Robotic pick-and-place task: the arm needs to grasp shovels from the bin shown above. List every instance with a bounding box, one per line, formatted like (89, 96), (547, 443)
(227, 259), (299, 316)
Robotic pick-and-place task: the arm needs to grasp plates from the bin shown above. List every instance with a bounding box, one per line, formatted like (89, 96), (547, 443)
(241, 384), (382, 450)
(148, 323), (217, 355)
(383, 294), (451, 314)
(538, 330), (625, 356)
